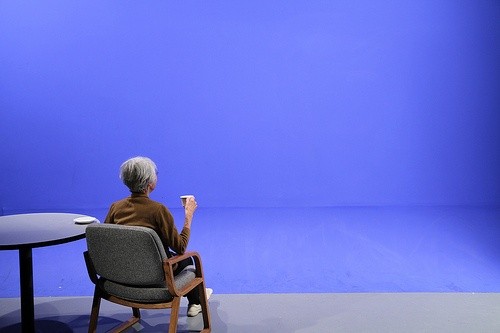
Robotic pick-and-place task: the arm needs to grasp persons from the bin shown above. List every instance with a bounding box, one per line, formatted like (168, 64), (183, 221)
(104, 156), (214, 318)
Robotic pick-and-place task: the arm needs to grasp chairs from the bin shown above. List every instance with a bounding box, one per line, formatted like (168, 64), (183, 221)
(81, 222), (213, 333)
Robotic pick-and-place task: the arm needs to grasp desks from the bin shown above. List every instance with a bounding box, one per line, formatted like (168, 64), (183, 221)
(0, 212), (100, 332)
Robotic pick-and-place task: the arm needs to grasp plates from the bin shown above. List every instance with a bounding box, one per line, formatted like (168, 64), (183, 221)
(74, 216), (97, 225)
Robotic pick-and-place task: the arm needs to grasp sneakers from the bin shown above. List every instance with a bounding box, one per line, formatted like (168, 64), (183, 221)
(187, 288), (213, 317)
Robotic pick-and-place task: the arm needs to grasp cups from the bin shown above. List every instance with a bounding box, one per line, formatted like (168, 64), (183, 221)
(179, 194), (195, 210)
